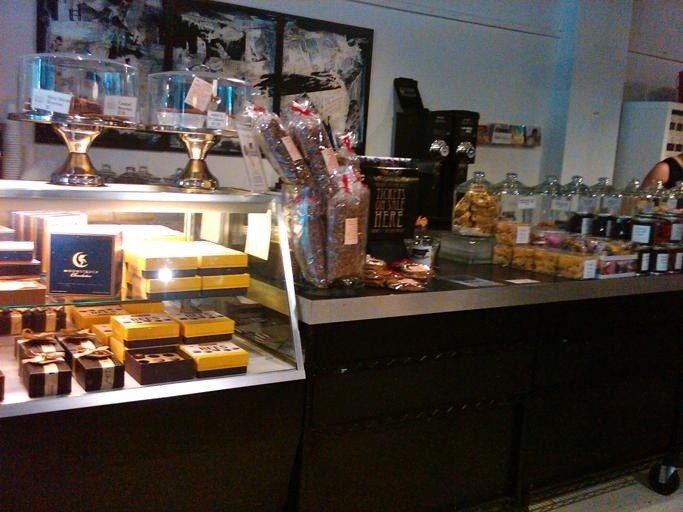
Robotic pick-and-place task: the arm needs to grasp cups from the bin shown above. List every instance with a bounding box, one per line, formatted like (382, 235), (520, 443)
(404, 232), (441, 267)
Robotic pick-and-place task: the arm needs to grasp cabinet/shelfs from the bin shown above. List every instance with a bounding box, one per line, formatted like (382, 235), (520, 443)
(0, 179), (308, 418)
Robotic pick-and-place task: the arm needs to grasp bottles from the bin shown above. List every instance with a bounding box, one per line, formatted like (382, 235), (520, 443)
(452, 171), (683, 234)
(98, 162), (183, 185)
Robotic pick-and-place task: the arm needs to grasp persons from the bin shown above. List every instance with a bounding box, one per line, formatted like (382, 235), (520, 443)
(527, 128), (540, 146)
(640, 153), (682, 210)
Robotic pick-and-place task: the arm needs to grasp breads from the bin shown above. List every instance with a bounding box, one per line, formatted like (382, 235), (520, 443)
(364, 259), (431, 293)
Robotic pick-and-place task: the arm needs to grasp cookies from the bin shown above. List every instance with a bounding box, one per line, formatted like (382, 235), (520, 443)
(454, 187), (497, 232)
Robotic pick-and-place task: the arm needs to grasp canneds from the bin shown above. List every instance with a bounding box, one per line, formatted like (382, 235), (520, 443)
(570, 210), (683, 275)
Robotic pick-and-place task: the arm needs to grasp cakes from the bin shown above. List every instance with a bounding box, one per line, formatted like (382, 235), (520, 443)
(155, 107), (237, 132)
(23, 92), (131, 123)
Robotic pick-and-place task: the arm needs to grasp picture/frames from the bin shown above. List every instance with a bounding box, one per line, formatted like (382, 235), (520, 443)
(35, 0), (375, 160)
